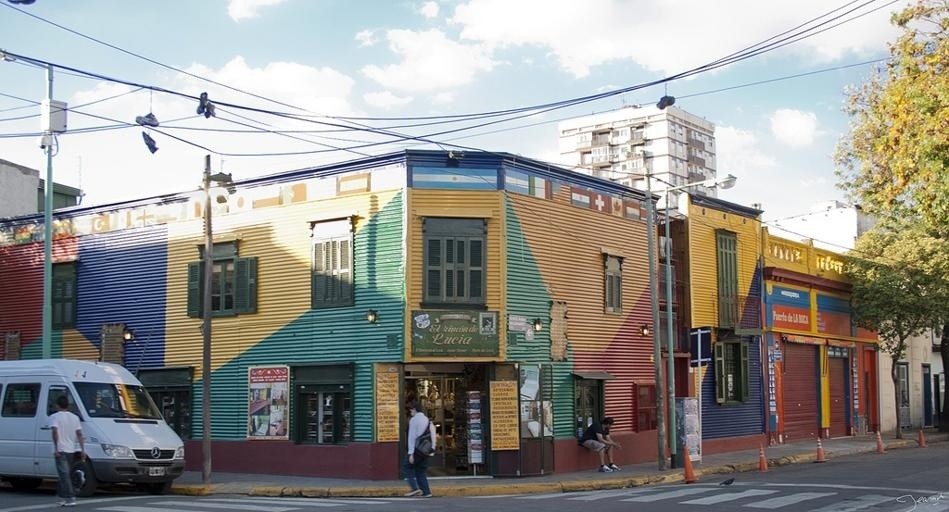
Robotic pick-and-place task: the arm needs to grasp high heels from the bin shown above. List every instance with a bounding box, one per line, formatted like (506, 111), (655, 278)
(874, 431), (887, 453)
(760, 443), (768, 472)
(919, 430), (928, 448)
(814, 437), (826, 461)
(682, 447), (699, 482)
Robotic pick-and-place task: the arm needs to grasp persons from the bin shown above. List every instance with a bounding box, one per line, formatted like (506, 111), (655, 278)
(49, 395), (87, 507)
(581, 417), (621, 473)
(403, 400), (437, 498)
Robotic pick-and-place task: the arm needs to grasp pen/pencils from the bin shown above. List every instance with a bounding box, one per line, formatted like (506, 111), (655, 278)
(0, 358), (186, 497)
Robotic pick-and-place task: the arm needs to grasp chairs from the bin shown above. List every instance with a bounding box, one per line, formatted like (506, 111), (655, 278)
(404, 488), (434, 499)
(56, 498), (75, 506)
(598, 463), (622, 473)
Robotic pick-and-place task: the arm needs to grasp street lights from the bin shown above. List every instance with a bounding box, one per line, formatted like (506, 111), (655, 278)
(413, 419), (435, 460)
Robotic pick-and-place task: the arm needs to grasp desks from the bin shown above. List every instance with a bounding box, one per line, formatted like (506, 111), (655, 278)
(665, 173), (737, 471)
(202, 154), (232, 484)
